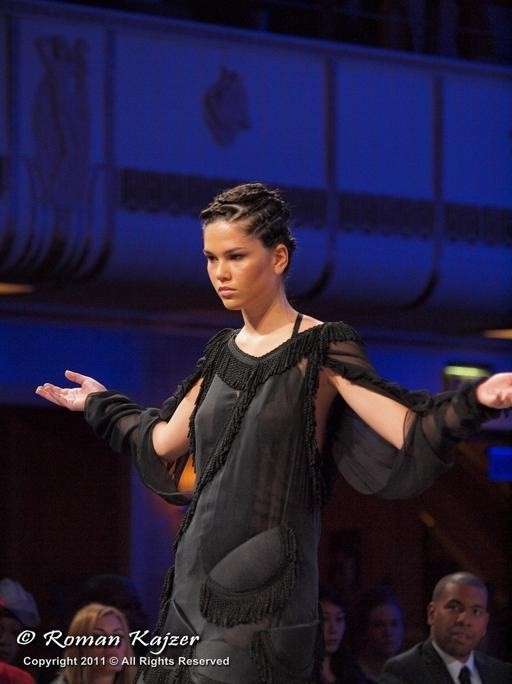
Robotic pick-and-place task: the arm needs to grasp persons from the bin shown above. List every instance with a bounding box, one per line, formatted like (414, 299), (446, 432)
(35, 183), (512, 683)
(0, 571), (512, 683)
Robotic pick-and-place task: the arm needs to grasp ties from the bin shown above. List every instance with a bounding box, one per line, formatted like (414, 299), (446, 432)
(459, 668), (471, 682)
